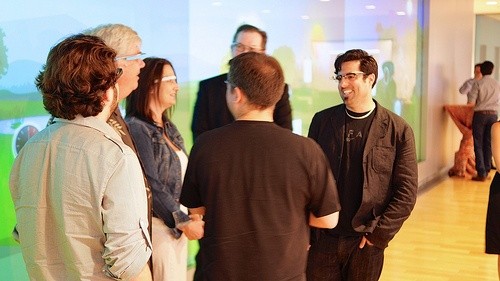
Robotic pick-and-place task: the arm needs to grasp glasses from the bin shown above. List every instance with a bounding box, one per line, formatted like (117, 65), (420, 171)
(152, 75), (177, 83)
(230, 42), (266, 55)
(222, 81), (237, 89)
(115, 52), (147, 62)
(332, 71), (370, 82)
(110, 68), (122, 85)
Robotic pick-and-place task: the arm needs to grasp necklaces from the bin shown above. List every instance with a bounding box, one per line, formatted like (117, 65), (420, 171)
(344, 104), (377, 120)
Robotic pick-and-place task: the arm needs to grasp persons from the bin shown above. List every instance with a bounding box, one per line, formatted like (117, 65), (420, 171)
(468, 60), (500, 181)
(84, 24), (151, 281)
(8, 34), (154, 281)
(191, 24), (294, 146)
(459, 64), (496, 170)
(307, 49), (418, 281)
(123, 58), (204, 281)
(179, 53), (341, 281)
(484, 120), (500, 281)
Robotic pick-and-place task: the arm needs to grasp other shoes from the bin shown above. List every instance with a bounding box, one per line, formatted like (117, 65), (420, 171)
(471, 176), (485, 182)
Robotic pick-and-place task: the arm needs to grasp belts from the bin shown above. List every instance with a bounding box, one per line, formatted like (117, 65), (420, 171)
(475, 110), (498, 115)
